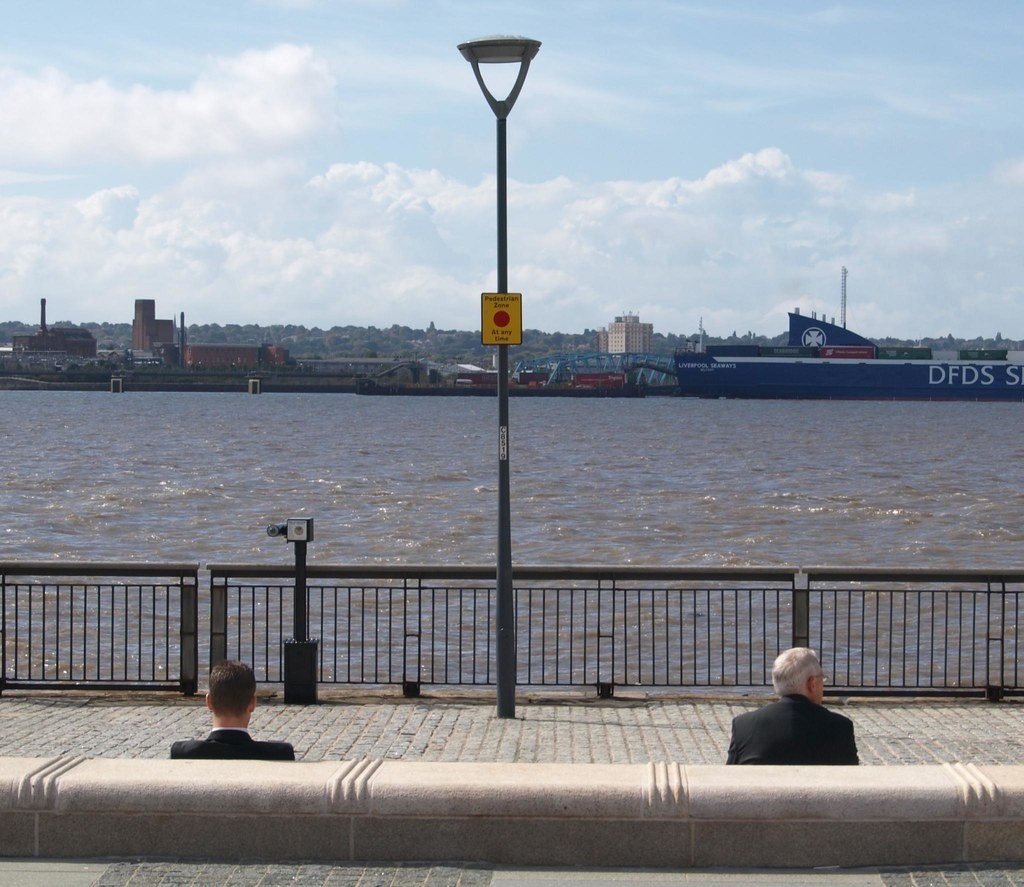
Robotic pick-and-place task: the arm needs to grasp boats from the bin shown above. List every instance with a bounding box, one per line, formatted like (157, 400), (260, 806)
(674, 307), (1024, 402)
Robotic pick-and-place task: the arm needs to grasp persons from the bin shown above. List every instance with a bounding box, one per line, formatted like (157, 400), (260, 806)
(170, 659), (295, 760)
(725, 648), (859, 765)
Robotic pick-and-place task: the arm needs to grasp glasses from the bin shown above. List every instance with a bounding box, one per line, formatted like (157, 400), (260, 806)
(813, 673), (828, 682)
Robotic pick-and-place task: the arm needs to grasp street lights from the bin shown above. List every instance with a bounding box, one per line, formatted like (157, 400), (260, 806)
(457, 35), (546, 721)
